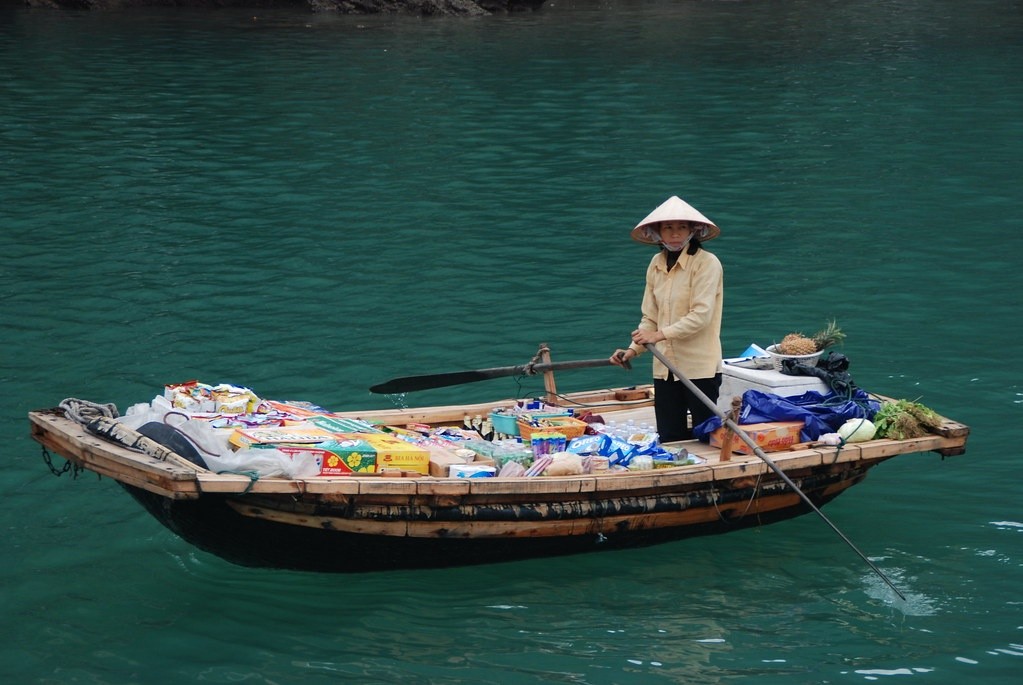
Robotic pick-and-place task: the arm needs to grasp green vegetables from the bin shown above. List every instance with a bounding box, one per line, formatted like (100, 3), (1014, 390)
(873, 398), (944, 441)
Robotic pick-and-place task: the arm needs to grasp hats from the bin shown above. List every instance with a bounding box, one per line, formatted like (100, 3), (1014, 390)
(630, 195), (720, 244)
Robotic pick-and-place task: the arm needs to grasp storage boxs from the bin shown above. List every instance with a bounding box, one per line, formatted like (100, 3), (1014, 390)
(151, 355), (909, 480)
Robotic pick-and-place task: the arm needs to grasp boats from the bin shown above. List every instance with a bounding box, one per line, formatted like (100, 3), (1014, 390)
(25, 377), (969, 572)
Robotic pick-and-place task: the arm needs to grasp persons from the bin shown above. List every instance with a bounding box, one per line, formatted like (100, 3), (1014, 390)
(610, 195), (725, 446)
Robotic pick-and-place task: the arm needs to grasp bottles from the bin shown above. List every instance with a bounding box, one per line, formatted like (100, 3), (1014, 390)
(464, 415), (514, 443)
(604, 419), (657, 441)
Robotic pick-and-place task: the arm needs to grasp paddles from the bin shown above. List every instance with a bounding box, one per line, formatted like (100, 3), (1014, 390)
(641, 343), (906, 601)
(369, 351), (633, 395)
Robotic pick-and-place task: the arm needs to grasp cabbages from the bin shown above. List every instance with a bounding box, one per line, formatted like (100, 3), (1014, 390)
(837, 418), (877, 443)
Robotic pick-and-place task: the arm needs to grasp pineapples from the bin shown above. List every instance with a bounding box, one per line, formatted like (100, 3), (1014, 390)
(778, 317), (847, 355)
